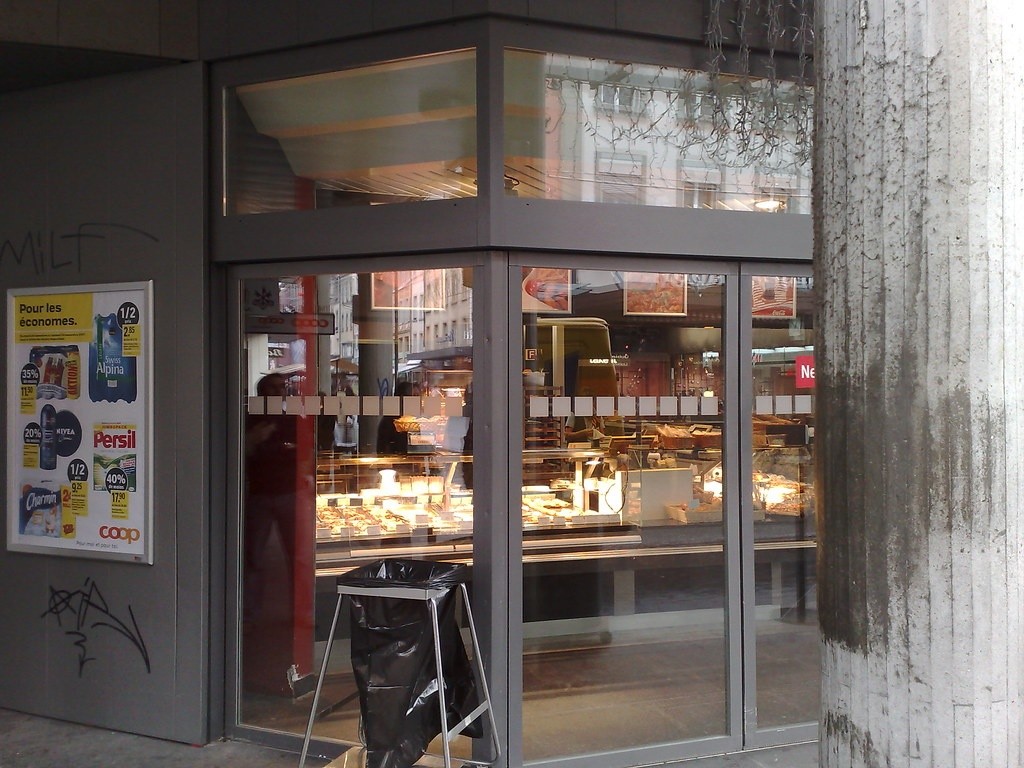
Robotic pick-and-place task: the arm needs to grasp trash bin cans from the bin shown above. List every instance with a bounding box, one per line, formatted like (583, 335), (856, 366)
(298, 558), (501, 768)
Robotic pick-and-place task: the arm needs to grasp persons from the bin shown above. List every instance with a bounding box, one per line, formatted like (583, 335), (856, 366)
(566, 387), (605, 471)
(377, 383), (420, 560)
(242, 373), (298, 604)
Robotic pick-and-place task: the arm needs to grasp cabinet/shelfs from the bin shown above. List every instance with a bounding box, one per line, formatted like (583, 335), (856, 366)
(316, 448), (820, 673)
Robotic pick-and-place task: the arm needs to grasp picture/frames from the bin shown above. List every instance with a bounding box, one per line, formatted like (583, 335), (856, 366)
(623, 271), (687, 316)
(751, 275), (797, 320)
(521, 266), (571, 314)
(371, 268), (446, 311)
